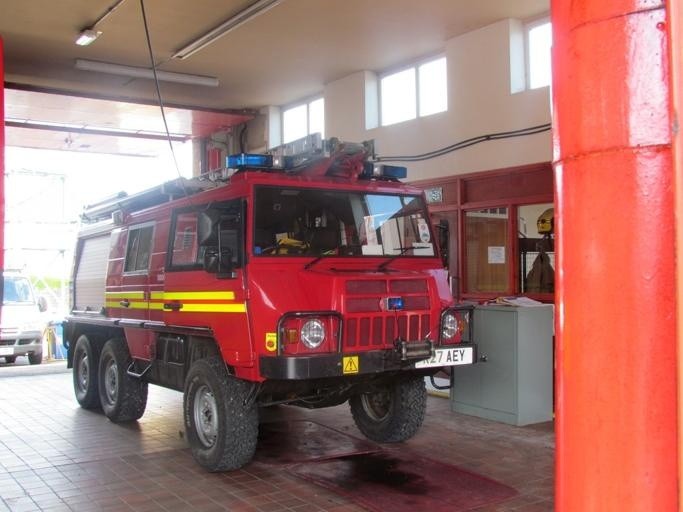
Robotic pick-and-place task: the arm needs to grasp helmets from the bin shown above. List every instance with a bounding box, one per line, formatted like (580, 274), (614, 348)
(537, 208), (554, 233)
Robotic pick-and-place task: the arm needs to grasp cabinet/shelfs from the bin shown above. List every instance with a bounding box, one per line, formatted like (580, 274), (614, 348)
(446, 302), (554, 428)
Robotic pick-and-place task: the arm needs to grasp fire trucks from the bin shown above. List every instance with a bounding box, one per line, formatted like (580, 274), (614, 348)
(58, 132), (477, 474)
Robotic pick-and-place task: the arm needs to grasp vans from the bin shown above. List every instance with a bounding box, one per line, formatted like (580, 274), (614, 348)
(0, 266), (45, 365)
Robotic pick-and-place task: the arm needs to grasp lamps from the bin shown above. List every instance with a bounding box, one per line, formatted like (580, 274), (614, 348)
(71, 58), (223, 89)
(74, 25), (102, 47)
